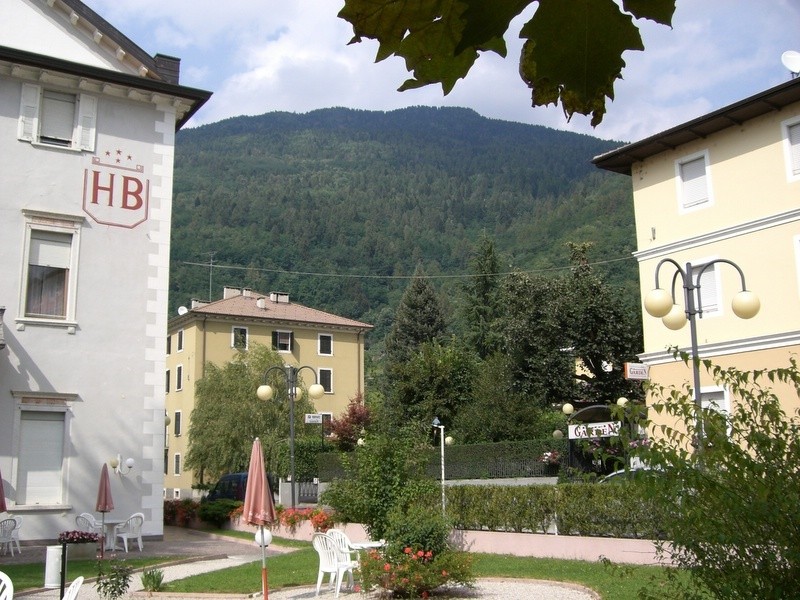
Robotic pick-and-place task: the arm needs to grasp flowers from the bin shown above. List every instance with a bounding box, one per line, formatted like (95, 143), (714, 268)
(540, 449), (561, 466)
(630, 438), (650, 448)
(58, 529), (101, 544)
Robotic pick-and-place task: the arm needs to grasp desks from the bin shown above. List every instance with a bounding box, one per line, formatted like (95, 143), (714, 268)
(92, 520), (126, 551)
(351, 542), (393, 556)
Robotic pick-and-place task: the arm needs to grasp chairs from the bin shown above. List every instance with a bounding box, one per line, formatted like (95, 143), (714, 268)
(62, 576), (85, 600)
(0, 571), (14, 600)
(0, 515), (23, 558)
(75, 512), (145, 553)
(327, 528), (364, 585)
(312, 533), (364, 600)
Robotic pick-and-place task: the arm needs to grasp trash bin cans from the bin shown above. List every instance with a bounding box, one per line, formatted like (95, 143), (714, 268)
(45, 544), (68, 589)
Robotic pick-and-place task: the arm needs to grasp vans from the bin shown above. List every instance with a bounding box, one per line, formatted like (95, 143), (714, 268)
(207, 472), (276, 506)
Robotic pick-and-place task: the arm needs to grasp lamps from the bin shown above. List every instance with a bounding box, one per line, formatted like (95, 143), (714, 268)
(110, 454), (135, 475)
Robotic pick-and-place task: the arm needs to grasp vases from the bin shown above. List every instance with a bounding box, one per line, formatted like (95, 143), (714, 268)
(67, 542), (96, 560)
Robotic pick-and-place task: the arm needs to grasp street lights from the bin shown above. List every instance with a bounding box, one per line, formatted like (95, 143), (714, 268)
(257, 365), (325, 509)
(643, 257), (760, 472)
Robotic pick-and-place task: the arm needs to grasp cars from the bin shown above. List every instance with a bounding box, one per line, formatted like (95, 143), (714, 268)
(598, 466), (668, 486)
